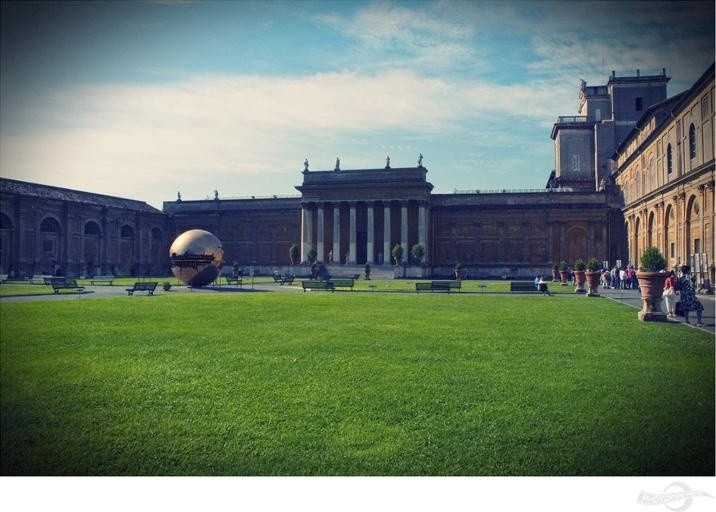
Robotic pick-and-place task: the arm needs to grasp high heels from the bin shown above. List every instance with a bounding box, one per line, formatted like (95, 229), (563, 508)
(696, 322), (704, 327)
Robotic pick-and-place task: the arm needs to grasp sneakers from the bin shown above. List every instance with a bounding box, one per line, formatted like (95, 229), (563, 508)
(668, 312), (676, 317)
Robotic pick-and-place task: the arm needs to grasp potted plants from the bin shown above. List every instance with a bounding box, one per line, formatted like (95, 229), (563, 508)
(454, 263), (462, 280)
(290, 244), (299, 265)
(307, 249), (318, 265)
(411, 243), (424, 266)
(558, 260), (568, 285)
(392, 243), (404, 265)
(572, 256), (587, 292)
(585, 257), (601, 297)
(634, 245), (668, 322)
(551, 265), (558, 282)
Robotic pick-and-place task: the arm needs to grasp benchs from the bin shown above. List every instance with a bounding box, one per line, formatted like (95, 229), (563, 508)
(432, 280), (461, 293)
(416, 283), (450, 294)
(0, 274), (85, 294)
(125, 282), (158, 295)
(511, 282), (547, 294)
(224, 273), (354, 293)
(89, 276), (115, 286)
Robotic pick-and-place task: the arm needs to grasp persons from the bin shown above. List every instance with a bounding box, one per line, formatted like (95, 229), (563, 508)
(534, 273), (541, 289)
(274, 268), (296, 285)
(447, 268), (470, 280)
(219, 263), (244, 285)
(566, 262), (696, 291)
(675, 265), (705, 327)
(538, 277), (555, 296)
(663, 271), (676, 316)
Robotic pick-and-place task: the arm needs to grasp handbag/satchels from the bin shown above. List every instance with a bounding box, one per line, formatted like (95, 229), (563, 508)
(663, 287), (675, 296)
